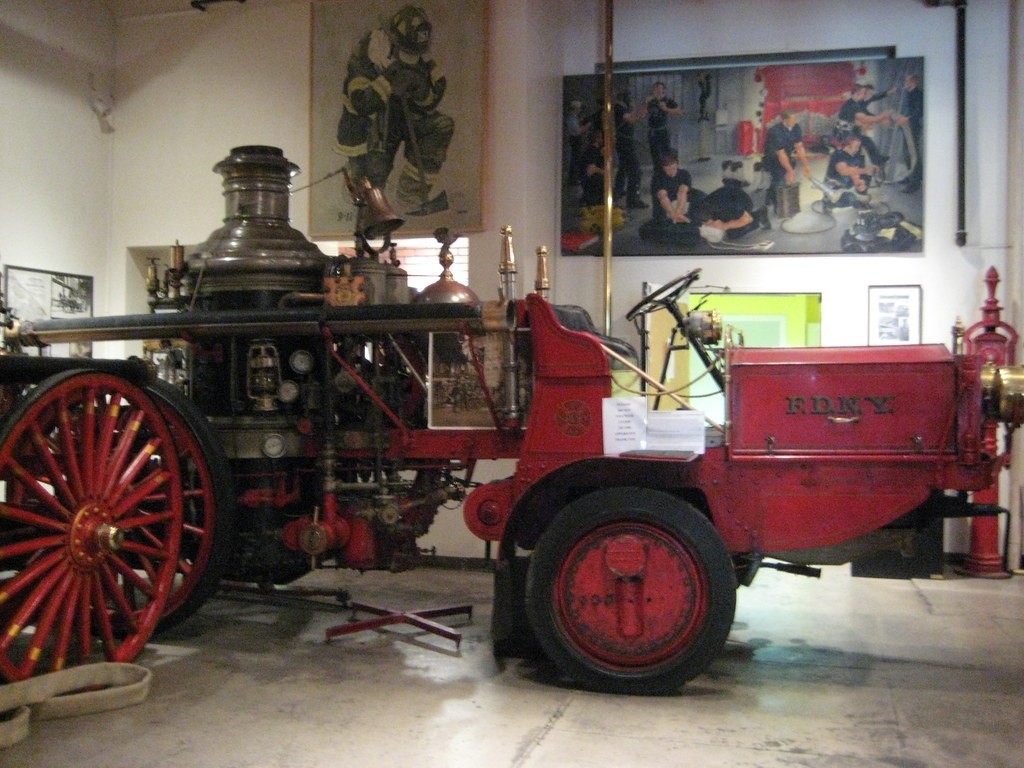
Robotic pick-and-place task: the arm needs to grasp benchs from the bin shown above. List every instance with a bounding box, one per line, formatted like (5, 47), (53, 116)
(526, 293), (645, 453)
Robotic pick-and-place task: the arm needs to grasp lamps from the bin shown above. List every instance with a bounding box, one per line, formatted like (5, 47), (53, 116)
(245, 336), (283, 412)
